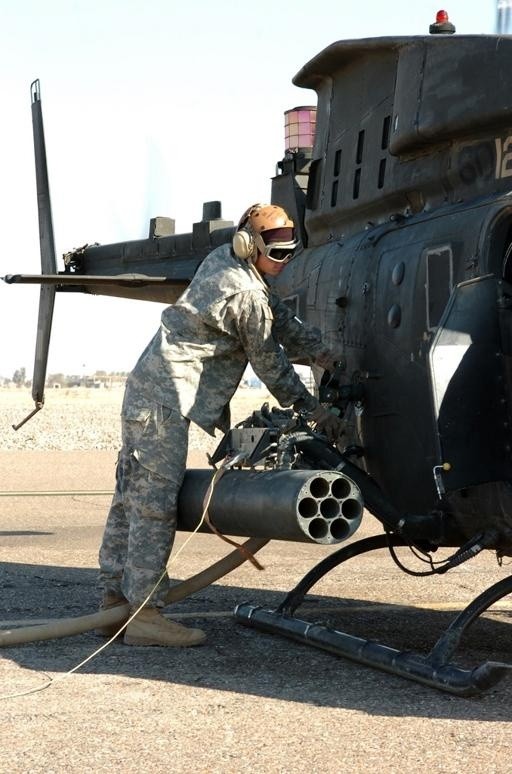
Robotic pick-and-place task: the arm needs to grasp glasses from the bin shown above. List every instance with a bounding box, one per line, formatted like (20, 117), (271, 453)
(259, 237), (301, 262)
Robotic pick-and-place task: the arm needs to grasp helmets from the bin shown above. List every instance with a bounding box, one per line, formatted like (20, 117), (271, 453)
(249, 204), (295, 234)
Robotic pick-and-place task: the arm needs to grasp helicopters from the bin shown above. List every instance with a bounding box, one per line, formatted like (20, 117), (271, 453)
(0, 6), (511, 705)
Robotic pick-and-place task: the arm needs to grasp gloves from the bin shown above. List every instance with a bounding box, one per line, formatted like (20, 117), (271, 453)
(315, 408), (343, 442)
(316, 350), (344, 371)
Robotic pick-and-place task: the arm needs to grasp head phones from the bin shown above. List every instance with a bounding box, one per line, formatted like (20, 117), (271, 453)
(232, 203), (262, 261)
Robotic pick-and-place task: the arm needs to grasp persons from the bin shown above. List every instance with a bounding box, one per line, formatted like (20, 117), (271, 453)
(94, 199), (364, 649)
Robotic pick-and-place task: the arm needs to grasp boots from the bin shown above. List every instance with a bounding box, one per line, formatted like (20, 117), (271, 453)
(93, 596), (133, 637)
(122, 602), (208, 647)
